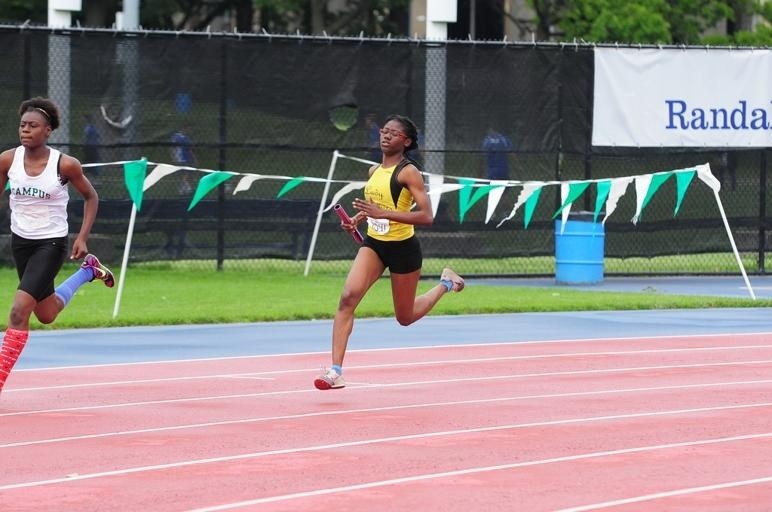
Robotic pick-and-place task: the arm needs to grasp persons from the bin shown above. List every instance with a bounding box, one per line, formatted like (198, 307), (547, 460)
(0, 95), (116, 390)
(314, 115), (464, 391)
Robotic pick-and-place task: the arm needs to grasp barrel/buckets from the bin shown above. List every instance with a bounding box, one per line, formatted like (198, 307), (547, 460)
(554, 211), (607, 286)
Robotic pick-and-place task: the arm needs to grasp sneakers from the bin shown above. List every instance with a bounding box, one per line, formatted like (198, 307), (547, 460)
(441, 268), (464, 292)
(81, 253), (114, 288)
(314, 369), (346, 390)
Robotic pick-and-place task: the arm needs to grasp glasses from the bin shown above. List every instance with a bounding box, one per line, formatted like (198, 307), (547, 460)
(379, 129), (407, 138)
